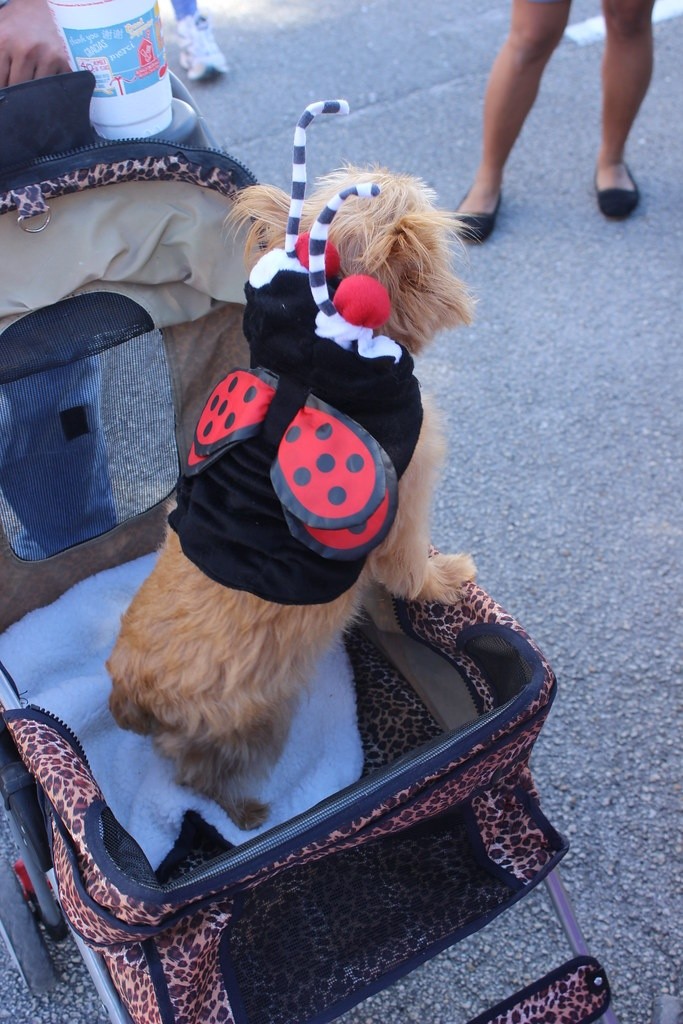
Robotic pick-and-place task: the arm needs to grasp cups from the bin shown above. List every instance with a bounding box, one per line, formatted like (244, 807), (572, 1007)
(47, 0), (172, 141)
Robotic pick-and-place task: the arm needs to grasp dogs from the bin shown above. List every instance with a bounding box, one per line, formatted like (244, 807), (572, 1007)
(100, 157), (478, 832)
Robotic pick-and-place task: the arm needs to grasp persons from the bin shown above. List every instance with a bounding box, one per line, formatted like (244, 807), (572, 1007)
(0, 1), (76, 90)
(454, 0), (654, 244)
(173, 1), (228, 81)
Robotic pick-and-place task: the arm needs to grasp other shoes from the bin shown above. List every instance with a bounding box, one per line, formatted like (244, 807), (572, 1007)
(449, 181), (502, 246)
(593, 163), (640, 222)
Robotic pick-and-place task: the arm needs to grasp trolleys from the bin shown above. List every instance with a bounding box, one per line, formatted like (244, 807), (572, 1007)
(0, 68), (620, 1024)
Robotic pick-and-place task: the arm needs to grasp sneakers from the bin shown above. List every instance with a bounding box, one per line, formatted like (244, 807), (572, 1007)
(178, 12), (228, 82)
